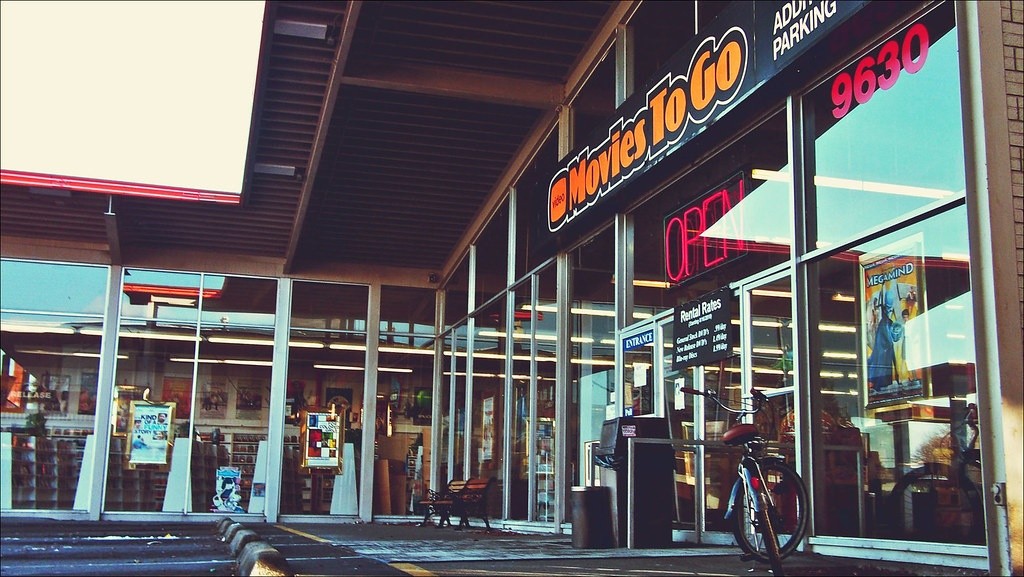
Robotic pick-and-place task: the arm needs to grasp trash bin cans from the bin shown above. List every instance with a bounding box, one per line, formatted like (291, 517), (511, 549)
(570, 485), (614, 549)
(594, 415), (676, 549)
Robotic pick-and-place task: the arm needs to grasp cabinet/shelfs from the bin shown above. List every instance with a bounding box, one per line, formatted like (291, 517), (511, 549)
(1, 408), (436, 515)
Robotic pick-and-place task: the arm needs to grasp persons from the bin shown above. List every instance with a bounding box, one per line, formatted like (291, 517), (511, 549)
(130, 410), (169, 453)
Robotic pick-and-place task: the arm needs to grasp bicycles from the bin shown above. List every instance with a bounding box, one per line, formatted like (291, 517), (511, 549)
(888, 402), (988, 547)
(678, 386), (810, 577)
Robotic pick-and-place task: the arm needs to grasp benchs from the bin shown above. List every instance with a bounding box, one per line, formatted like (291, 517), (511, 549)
(416, 475), (498, 531)
